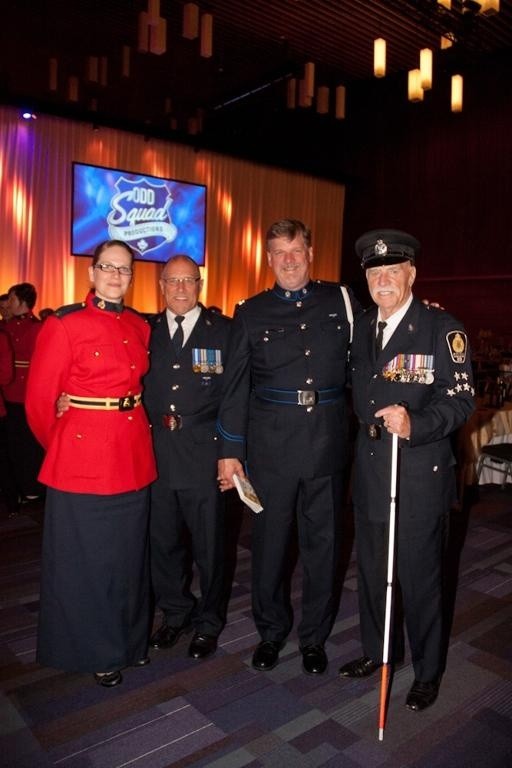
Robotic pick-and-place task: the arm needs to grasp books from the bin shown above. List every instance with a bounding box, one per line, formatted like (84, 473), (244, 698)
(232, 472), (265, 515)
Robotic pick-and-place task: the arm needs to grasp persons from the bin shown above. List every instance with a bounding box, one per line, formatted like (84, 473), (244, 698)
(53, 253), (239, 660)
(38, 307), (54, 321)
(23, 240), (161, 689)
(336, 227), (483, 712)
(2, 291), (14, 323)
(0, 330), (15, 415)
(216, 217), (446, 676)
(1, 281), (43, 511)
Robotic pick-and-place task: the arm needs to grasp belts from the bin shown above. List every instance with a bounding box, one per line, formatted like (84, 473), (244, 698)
(155, 407), (217, 433)
(66, 392), (142, 411)
(359, 420), (392, 441)
(261, 387), (342, 407)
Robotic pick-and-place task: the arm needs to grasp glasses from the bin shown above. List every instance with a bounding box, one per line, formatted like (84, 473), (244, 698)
(94, 263), (133, 275)
(162, 277), (200, 287)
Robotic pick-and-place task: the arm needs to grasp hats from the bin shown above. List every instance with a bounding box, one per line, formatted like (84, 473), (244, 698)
(355, 228), (421, 271)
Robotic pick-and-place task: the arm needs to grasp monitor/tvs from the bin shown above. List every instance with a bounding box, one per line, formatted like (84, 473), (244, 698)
(71, 160), (207, 267)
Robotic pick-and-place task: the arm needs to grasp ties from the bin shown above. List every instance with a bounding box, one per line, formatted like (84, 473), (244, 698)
(376, 322), (387, 359)
(171, 316), (184, 359)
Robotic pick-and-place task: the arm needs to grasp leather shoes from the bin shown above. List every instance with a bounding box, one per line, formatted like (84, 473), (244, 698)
(7, 494), (45, 507)
